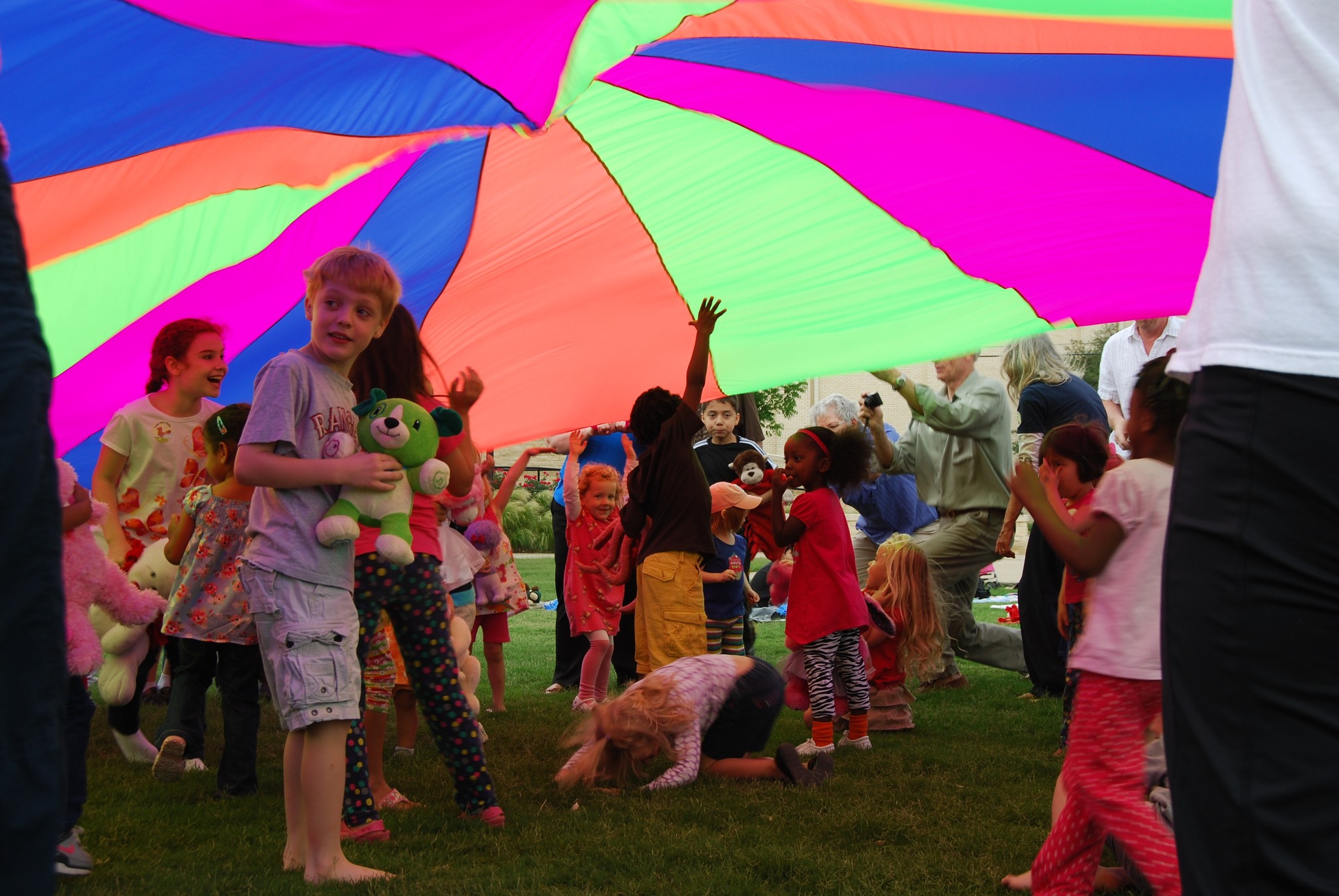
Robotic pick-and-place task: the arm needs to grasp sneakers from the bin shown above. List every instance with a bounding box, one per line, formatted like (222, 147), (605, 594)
(137, 687), (159, 702)
(151, 737), (186, 783)
(793, 738), (835, 756)
(155, 687), (172, 704)
(775, 744), (821, 786)
(391, 750), (414, 765)
(52, 837), (95, 875)
(838, 730), (873, 750)
(808, 751), (834, 781)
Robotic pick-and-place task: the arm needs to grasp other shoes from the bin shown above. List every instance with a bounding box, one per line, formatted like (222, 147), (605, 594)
(573, 696), (599, 711)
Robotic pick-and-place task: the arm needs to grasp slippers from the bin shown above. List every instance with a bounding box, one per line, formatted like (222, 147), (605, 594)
(374, 788), (427, 811)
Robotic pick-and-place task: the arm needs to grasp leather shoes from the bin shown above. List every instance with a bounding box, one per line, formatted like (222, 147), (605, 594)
(912, 670), (971, 694)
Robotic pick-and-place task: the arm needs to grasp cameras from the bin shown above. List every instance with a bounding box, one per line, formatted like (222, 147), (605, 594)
(863, 391), (883, 409)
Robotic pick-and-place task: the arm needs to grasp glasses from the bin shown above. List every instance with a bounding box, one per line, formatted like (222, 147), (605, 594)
(827, 420), (845, 431)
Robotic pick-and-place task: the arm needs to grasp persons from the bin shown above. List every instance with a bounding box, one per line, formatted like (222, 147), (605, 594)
(0, 4), (1339, 896)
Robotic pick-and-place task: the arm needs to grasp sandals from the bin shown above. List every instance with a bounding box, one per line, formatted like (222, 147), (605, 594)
(336, 821), (392, 843)
(455, 807), (507, 827)
